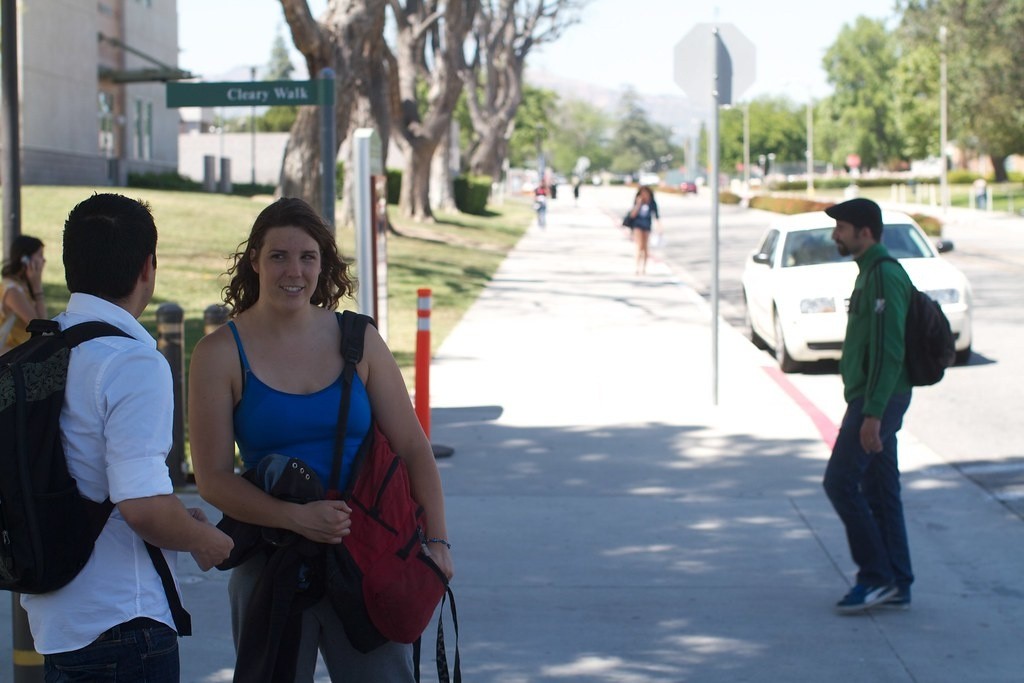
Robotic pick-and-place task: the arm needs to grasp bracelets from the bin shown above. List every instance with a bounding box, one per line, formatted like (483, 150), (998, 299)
(424, 537), (451, 550)
(32, 290), (45, 301)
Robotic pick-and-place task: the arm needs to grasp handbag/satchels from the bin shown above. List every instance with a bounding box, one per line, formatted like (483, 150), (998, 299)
(624, 212), (633, 228)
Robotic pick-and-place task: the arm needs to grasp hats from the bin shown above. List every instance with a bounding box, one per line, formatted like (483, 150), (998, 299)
(826, 198), (881, 225)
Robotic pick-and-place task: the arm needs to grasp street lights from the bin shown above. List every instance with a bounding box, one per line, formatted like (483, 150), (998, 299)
(937, 25), (951, 207)
(721, 104), (750, 207)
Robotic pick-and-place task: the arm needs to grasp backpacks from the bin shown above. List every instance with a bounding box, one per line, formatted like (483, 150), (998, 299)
(0, 317), (137, 594)
(329, 428), (455, 645)
(871, 257), (955, 385)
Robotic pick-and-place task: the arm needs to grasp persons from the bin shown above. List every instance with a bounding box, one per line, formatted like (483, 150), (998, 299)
(573, 178), (582, 202)
(547, 176), (558, 199)
(19, 189), (234, 683)
(192, 198), (455, 683)
(821, 200), (915, 617)
(630, 185), (660, 276)
(973, 176), (987, 211)
(533, 178), (550, 227)
(0, 234), (48, 359)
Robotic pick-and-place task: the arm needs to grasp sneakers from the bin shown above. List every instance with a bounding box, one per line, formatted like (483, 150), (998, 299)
(835, 576), (912, 613)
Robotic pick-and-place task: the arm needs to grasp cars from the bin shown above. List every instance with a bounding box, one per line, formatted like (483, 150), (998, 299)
(639, 172), (661, 186)
(741, 209), (973, 374)
(680, 182), (696, 194)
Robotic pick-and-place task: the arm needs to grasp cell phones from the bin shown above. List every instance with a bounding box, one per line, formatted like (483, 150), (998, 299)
(20, 255), (30, 266)
